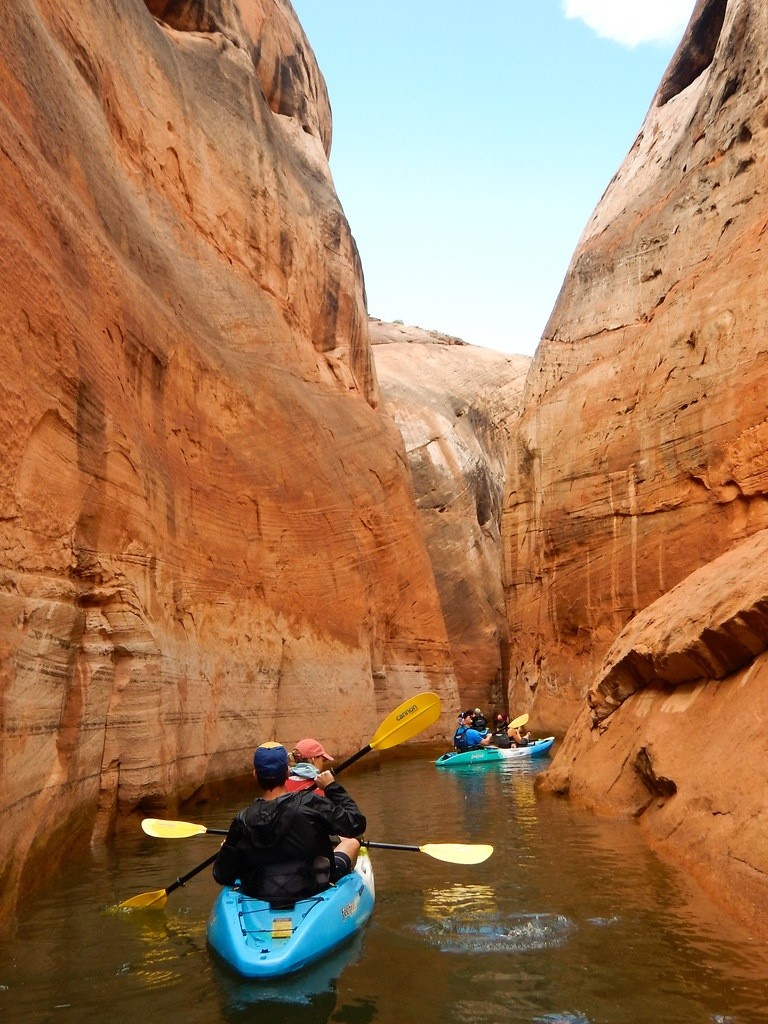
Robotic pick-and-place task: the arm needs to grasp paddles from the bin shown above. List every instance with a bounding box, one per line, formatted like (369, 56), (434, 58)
(115, 690), (441, 906)
(492, 714), (530, 737)
(139, 814), (495, 865)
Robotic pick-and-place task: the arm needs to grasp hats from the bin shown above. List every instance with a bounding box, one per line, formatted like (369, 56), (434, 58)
(496, 713), (508, 728)
(254, 741), (289, 769)
(456, 708), (473, 725)
(292, 738), (335, 762)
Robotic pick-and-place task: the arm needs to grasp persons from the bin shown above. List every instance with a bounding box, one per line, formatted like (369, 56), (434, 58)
(453, 707), (532, 754)
(213, 739), (366, 899)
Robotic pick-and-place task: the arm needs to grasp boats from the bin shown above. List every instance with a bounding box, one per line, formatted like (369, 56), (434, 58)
(435, 736), (556, 766)
(206, 841), (375, 979)
